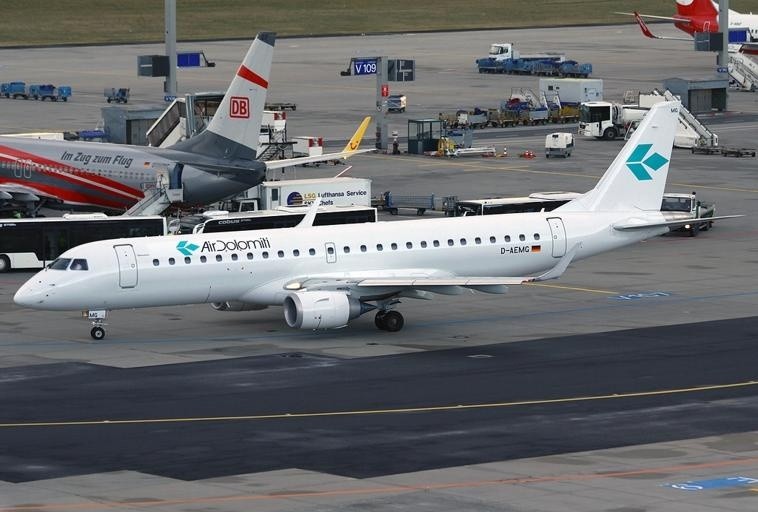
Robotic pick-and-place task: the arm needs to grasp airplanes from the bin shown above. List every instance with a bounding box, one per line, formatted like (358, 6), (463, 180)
(613, 1), (758, 56)
